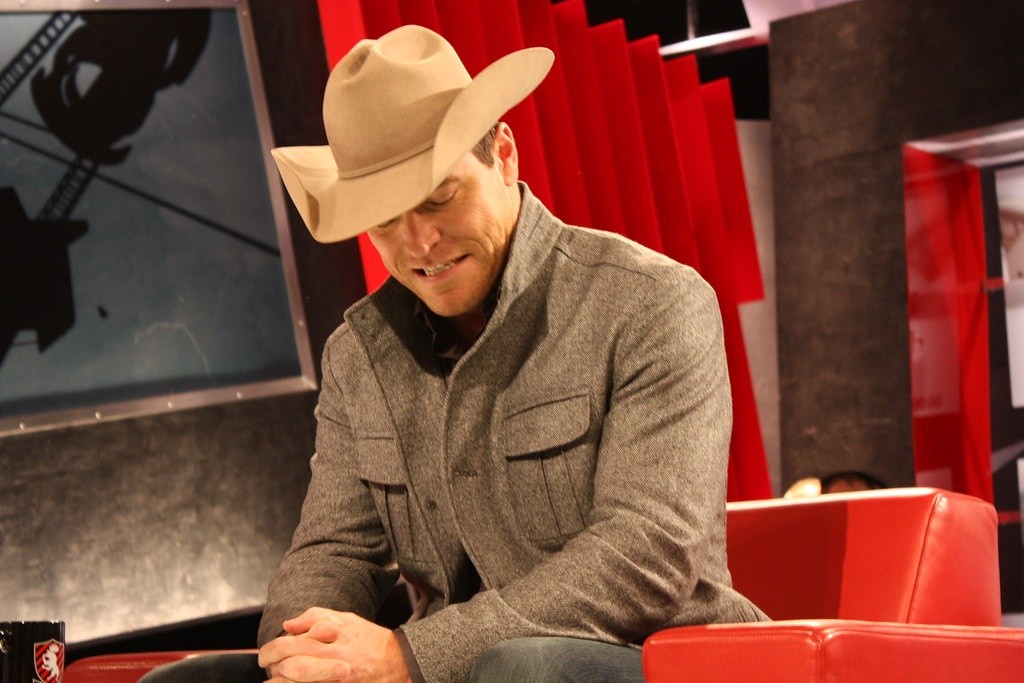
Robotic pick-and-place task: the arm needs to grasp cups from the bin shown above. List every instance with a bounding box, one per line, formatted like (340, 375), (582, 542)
(0, 620), (66, 683)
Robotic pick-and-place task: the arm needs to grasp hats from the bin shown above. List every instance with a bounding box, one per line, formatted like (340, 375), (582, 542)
(269, 26), (556, 244)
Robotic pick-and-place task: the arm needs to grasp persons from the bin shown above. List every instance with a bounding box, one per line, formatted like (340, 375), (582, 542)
(260, 25), (772, 683)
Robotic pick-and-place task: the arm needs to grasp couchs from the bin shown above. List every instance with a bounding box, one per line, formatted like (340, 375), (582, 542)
(66, 486), (1024, 683)
